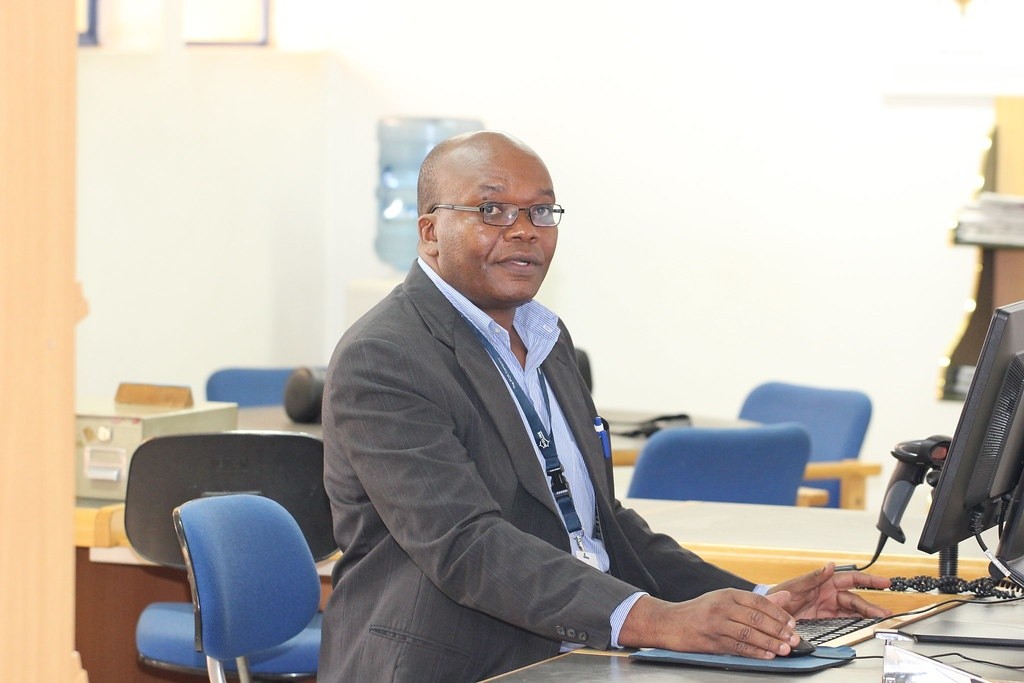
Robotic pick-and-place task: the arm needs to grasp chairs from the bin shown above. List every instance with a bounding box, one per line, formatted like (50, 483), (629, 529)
(123, 433), (340, 683)
(171, 492), (319, 682)
(207, 368), (293, 407)
(623, 428), (829, 507)
(736, 382), (884, 510)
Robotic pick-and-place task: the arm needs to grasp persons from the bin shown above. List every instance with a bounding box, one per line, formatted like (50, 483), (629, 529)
(315, 131), (891, 683)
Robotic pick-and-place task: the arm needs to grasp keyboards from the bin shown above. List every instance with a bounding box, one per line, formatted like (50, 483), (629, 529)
(787, 615), (884, 646)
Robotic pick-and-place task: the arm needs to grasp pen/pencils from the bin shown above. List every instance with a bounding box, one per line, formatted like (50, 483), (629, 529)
(594, 416), (611, 459)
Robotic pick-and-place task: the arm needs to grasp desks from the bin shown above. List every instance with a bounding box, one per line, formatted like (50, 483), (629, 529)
(92, 437), (1024, 682)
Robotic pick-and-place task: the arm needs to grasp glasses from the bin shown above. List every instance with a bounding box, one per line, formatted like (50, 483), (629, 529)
(429, 202), (564, 227)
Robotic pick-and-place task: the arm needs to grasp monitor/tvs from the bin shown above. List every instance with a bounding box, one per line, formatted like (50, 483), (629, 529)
(917, 300), (1024, 591)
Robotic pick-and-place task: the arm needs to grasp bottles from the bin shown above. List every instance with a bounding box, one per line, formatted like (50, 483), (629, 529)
(376, 116), (486, 273)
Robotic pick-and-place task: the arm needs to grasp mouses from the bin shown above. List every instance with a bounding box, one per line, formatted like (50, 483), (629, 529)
(775, 636), (816, 658)
